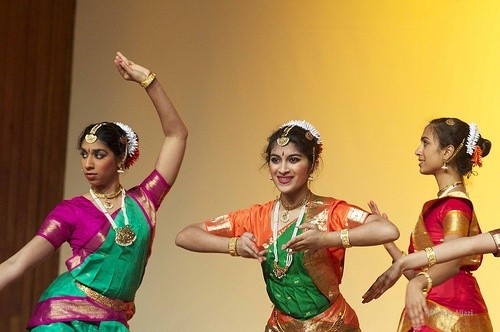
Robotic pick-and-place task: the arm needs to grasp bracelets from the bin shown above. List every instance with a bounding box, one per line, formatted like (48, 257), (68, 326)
(416, 272), (432, 293)
(140, 71), (157, 89)
(340, 228), (352, 249)
(228, 237), (240, 257)
(423, 247), (436, 267)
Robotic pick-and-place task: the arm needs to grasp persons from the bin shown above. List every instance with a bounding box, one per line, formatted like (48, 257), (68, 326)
(362, 228), (500, 304)
(371, 116), (493, 332)
(0, 51), (188, 332)
(173, 119), (399, 332)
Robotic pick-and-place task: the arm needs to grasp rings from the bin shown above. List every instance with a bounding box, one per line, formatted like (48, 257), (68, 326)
(304, 249), (309, 253)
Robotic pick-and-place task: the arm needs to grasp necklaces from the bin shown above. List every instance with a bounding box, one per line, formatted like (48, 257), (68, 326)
(90, 186), (137, 247)
(279, 190), (310, 221)
(269, 195), (307, 280)
(93, 186), (124, 209)
(435, 181), (465, 196)
